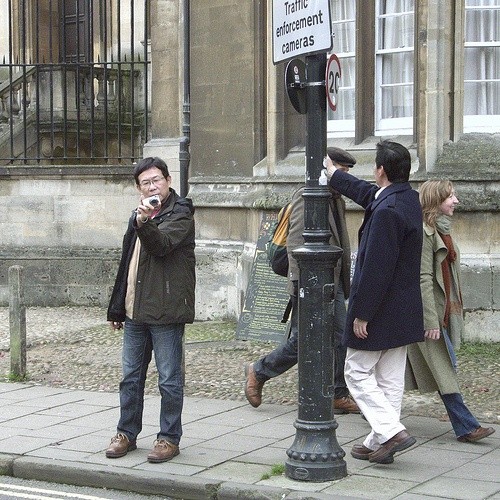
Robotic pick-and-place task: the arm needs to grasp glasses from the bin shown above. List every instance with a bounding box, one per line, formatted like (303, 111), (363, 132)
(138, 176), (166, 188)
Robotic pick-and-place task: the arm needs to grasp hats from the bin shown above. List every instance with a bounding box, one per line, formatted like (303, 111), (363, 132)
(327, 147), (356, 168)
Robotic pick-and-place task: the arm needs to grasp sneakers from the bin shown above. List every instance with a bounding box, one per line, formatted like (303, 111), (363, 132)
(106, 434), (137, 457)
(147, 439), (180, 462)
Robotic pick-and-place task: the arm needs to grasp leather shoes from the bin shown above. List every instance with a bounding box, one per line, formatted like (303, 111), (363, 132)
(369, 430), (416, 463)
(457, 426), (495, 442)
(333, 396), (361, 414)
(351, 444), (393, 463)
(245, 360), (263, 408)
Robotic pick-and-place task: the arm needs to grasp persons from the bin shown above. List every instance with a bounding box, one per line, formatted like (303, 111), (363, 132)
(323, 138), (425, 464)
(404, 178), (495, 444)
(107, 157), (196, 464)
(245, 147), (361, 415)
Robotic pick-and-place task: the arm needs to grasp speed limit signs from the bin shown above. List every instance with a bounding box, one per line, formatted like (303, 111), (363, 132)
(325, 53), (341, 112)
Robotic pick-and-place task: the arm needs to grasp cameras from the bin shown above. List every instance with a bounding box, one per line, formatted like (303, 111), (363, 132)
(141, 195), (161, 210)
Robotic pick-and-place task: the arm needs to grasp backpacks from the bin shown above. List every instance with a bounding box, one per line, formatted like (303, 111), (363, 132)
(269, 186), (305, 277)
